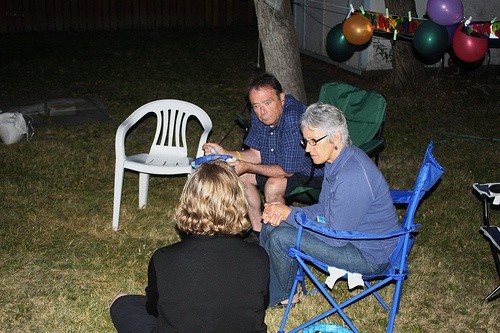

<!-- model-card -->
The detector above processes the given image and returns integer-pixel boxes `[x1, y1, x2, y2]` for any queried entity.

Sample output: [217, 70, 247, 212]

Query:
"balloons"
[452, 24, 488, 62]
[414, 20, 448, 57]
[326, 23, 356, 62]
[427, 0, 463, 26]
[343, 15, 373, 45]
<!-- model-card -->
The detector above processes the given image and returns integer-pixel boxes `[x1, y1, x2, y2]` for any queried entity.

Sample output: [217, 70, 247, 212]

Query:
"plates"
[191, 155, 240, 170]
[302, 323, 355, 333]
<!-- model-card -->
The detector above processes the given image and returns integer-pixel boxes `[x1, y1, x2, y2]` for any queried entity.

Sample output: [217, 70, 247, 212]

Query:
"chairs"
[111, 98, 213, 233]
[220, 80, 389, 240]
[472, 182, 500, 301]
[278, 140, 445, 333]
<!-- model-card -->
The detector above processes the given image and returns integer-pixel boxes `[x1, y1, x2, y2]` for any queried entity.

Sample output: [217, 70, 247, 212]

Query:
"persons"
[109, 159, 270, 333]
[259, 102, 403, 308]
[202, 74, 324, 233]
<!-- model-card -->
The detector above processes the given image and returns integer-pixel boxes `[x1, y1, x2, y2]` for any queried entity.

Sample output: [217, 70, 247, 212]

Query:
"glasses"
[300, 134, 327, 146]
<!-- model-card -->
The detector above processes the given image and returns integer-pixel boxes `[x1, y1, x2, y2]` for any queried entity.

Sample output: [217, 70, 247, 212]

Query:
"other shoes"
[273, 299, 300, 309]
